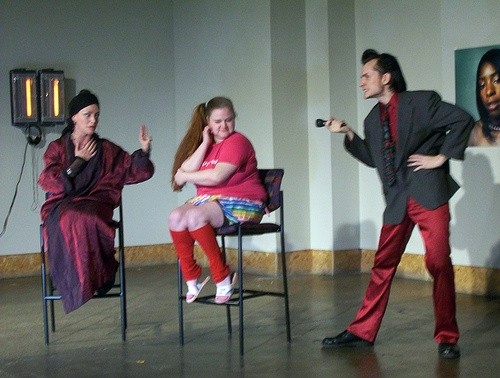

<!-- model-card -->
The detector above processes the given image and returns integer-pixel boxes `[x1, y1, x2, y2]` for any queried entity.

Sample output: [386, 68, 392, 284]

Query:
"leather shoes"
[438, 343, 459, 360]
[320, 329, 374, 348]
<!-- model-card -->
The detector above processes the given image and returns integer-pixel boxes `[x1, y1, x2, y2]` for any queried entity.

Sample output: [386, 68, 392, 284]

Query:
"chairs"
[38, 188, 128, 345]
[177, 167, 293, 359]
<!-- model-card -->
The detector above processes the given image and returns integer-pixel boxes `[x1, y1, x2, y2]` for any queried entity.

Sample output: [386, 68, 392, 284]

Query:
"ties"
[383, 103, 396, 188]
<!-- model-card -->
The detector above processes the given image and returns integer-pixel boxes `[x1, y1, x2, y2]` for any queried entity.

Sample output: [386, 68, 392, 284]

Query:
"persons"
[320, 48, 479, 359]
[167, 95, 267, 306]
[35, 90, 155, 316]
[464, 47, 500, 148]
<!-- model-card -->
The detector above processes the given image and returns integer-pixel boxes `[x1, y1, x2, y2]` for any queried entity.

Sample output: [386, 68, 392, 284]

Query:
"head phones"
[24, 125, 42, 146]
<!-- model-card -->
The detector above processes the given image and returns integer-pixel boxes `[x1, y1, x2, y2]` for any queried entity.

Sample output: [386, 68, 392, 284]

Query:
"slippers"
[214, 271, 238, 303]
[185, 275, 210, 304]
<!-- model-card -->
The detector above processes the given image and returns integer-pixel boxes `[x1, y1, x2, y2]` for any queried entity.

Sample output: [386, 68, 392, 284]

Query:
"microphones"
[316, 118, 347, 127]
